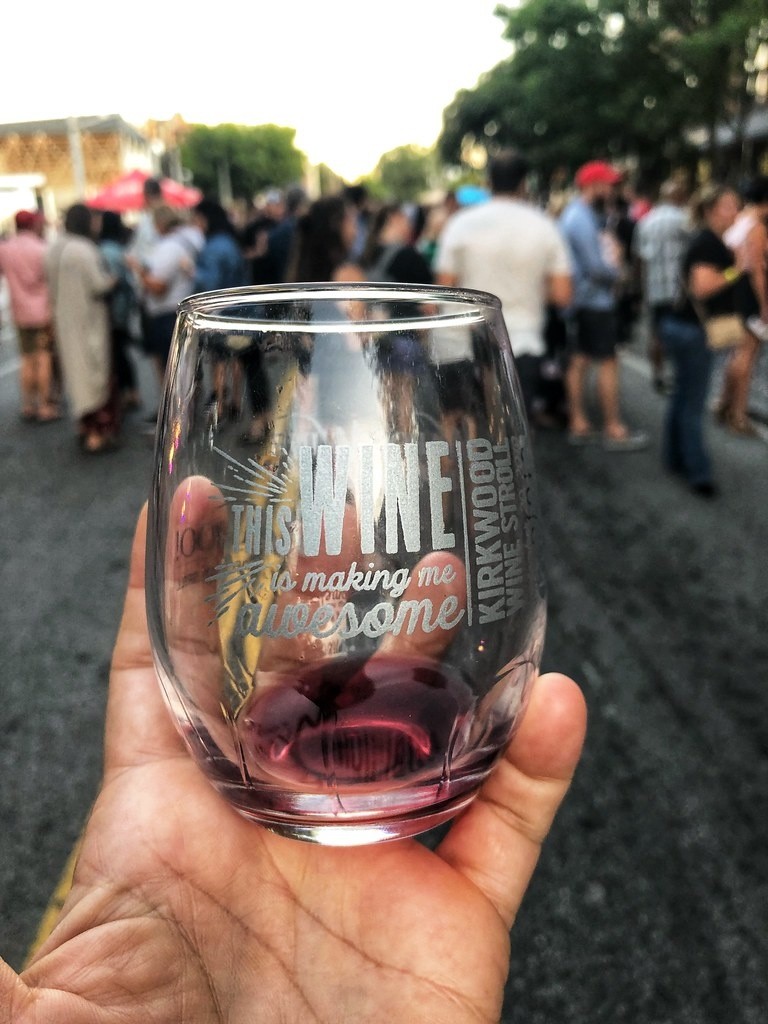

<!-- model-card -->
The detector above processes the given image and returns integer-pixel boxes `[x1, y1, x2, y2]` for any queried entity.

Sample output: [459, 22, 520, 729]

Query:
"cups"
[144, 280, 546, 848]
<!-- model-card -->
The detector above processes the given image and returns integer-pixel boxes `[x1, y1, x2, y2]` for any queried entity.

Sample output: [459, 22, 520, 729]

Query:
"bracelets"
[723, 266, 738, 282]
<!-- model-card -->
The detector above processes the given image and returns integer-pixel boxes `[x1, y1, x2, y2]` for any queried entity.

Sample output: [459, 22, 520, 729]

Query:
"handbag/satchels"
[702, 316, 747, 347]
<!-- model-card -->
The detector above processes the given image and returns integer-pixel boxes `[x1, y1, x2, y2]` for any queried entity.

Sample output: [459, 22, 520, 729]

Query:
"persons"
[1, 473, 590, 1024]
[0, 146, 768, 500]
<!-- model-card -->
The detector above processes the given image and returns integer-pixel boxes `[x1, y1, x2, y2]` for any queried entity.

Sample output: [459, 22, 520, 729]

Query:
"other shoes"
[608, 432, 647, 451]
[568, 431, 583, 445]
[20, 380, 270, 453]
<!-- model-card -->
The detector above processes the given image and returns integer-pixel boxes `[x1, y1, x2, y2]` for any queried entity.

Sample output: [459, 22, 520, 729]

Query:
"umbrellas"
[86, 170, 204, 212]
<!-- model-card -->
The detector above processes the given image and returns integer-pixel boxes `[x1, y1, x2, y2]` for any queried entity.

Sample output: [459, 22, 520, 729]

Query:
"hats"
[16, 213, 36, 225]
[576, 163, 620, 185]
